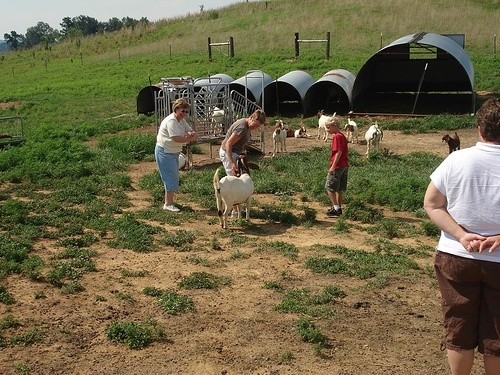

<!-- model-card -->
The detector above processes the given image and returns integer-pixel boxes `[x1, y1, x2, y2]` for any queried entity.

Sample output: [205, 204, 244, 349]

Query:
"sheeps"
[317, 110, 336, 142]
[364, 125, 384, 153]
[212, 149, 255, 230]
[208, 105, 237, 138]
[271, 119, 315, 158]
[344, 120, 360, 144]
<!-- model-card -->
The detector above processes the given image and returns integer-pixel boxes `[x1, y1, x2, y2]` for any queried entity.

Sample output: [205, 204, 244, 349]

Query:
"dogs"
[442, 132, 460, 153]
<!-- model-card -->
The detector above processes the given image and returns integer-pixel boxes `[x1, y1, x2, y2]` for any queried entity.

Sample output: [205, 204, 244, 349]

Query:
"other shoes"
[326, 208, 342, 215]
[163, 203, 181, 212]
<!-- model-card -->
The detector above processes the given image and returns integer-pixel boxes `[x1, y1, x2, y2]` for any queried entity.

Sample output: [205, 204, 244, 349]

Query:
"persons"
[155, 98, 195, 212]
[325, 117, 348, 217]
[219, 109, 266, 219]
[424, 98, 500, 375]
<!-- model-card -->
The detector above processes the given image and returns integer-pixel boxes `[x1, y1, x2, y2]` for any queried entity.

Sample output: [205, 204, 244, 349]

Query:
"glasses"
[179, 109, 188, 114]
